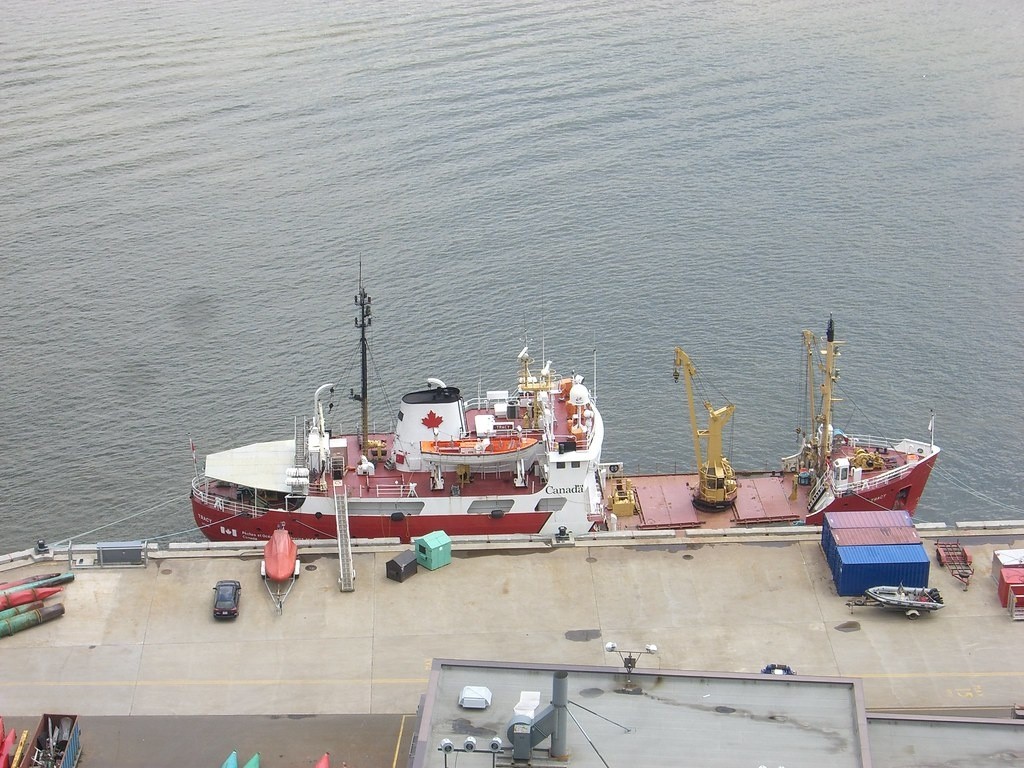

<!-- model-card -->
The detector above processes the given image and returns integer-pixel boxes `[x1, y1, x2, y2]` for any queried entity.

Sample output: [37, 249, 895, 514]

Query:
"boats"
[419, 438, 540, 467]
[191, 254, 943, 540]
[264, 529, 298, 581]
[865, 578, 946, 612]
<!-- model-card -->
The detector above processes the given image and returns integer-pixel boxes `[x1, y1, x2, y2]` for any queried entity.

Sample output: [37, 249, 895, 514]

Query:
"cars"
[213, 579, 242, 620]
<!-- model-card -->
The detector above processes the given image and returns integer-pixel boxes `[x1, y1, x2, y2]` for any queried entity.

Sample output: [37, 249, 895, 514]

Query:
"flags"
[190, 440, 197, 463]
[928, 420, 932, 434]
